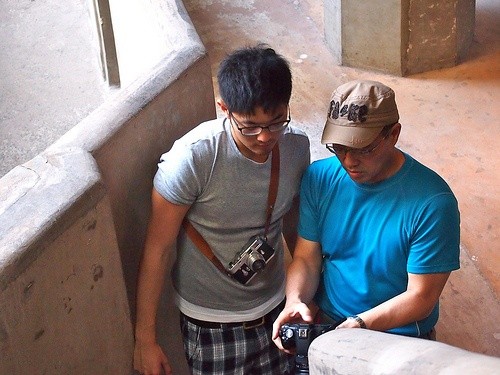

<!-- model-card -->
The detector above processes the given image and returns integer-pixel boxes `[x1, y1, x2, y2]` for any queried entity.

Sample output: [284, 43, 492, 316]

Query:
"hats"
[321, 80, 399, 148]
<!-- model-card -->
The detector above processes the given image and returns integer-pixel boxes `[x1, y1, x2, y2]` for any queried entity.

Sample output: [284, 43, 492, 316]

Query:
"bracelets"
[352, 315, 367, 329]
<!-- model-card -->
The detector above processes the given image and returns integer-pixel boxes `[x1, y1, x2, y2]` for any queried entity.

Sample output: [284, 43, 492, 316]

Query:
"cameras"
[279, 323, 336, 375]
[226, 235, 276, 286]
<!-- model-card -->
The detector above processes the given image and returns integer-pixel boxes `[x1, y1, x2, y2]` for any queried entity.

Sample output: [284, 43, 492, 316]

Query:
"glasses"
[325, 134, 385, 156]
[227, 104, 291, 135]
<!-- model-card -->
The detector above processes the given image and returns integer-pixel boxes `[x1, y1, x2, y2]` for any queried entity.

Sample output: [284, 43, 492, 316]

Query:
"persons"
[134, 41, 319, 375]
[272, 79, 460, 355]
